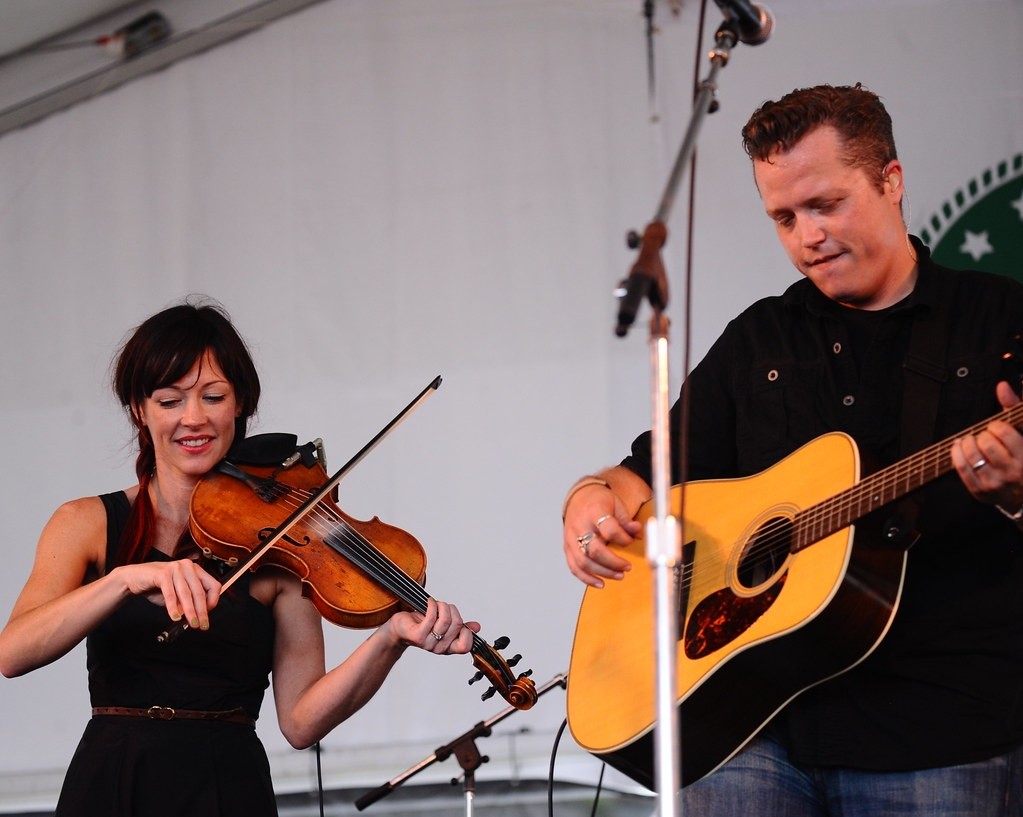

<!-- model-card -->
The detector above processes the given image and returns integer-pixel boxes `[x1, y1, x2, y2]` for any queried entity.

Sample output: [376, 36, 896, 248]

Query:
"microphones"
[715, 0, 773, 47]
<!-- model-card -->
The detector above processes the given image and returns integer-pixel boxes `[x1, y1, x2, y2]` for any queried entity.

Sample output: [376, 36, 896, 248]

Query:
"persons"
[0, 304, 480, 817]
[563, 84, 1023, 817]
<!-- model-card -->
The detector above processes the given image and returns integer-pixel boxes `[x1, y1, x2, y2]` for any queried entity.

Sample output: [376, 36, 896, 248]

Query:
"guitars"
[566, 401, 1022, 795]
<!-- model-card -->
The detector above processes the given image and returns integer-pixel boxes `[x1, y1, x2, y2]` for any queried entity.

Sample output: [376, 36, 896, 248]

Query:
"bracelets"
[562, 479, 612, 524]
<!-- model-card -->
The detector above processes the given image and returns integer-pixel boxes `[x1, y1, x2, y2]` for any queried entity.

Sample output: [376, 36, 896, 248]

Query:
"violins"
[186, 432, 539, 712]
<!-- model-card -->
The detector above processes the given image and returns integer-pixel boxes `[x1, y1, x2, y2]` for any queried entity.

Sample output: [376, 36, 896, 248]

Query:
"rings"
[577, 533, 597, 554]
[430, 630, 444, 640]
[972, 460, 986, 470]
[596, 515, 611, 527]
